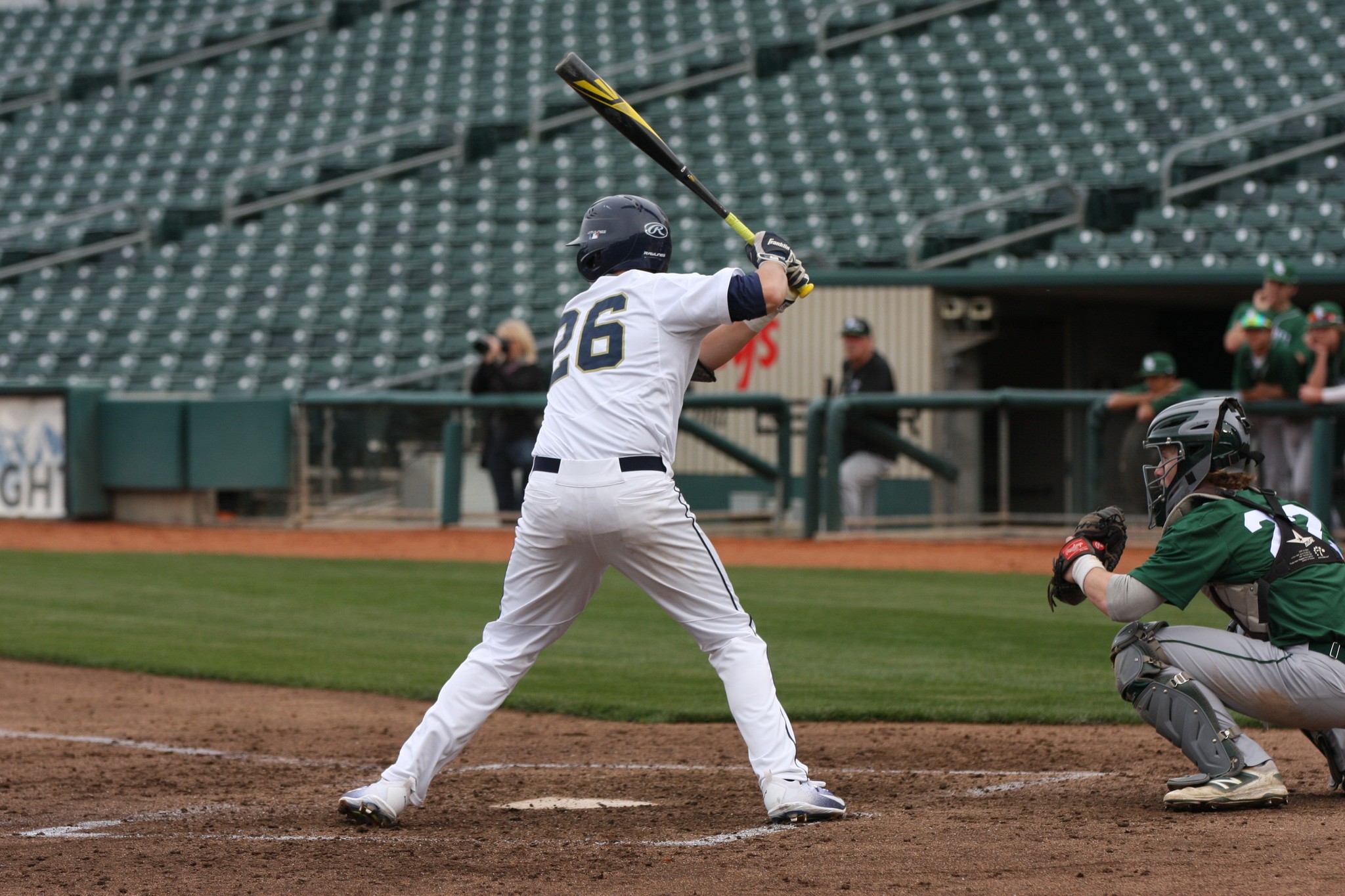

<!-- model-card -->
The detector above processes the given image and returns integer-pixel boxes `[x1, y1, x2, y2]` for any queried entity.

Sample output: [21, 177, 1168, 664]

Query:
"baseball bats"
[553, 50, 816, 300]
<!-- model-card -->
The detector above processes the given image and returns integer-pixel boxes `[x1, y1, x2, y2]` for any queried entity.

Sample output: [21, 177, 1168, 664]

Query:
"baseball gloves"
[1052, 505, 1129, 606]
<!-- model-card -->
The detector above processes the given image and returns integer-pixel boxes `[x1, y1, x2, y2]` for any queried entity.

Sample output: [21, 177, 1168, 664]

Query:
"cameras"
[474, 338, 511, 356]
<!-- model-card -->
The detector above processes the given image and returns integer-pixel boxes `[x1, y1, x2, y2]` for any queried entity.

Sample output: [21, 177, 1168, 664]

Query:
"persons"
[1063, 396, 1345, 809]
[469, 318, 542, 526]
[819, 315, 901, 533]
[1087, 258, 1345, 511]
[336, 194, 847, 828]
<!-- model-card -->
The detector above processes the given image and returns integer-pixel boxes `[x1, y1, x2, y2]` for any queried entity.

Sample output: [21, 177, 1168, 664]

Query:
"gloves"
[745, 232, 790, 271]
[742, 257, 808, 334]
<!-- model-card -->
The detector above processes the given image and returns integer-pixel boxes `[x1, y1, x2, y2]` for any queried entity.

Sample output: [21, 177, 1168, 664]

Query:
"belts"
[532, 456, 668, 474]
[1309, 640, 1345, 663]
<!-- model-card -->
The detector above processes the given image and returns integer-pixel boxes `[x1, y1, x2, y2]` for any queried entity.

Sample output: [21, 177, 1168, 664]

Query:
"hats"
[1242, 312, 1270, 330]
[842, 319, 872, 336]
[1264, 259, 1300, 286]
[1304, 301, 1344, 329]
[1140, 352, 1173, 377]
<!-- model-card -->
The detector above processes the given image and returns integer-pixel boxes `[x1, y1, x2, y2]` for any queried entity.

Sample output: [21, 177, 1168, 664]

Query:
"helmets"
[564, 194, 671, 281]
[1140, 397, 1251, 530]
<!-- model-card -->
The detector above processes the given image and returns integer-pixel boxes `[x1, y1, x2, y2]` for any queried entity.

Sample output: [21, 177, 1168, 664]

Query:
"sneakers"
[761, 769, 847, 822]
[1163, 759, 1289, 811]
[337, 778, 418, 828]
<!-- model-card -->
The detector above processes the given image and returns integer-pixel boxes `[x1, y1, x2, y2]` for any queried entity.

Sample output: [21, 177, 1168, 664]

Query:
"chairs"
[0, 0, 1345, 397]
